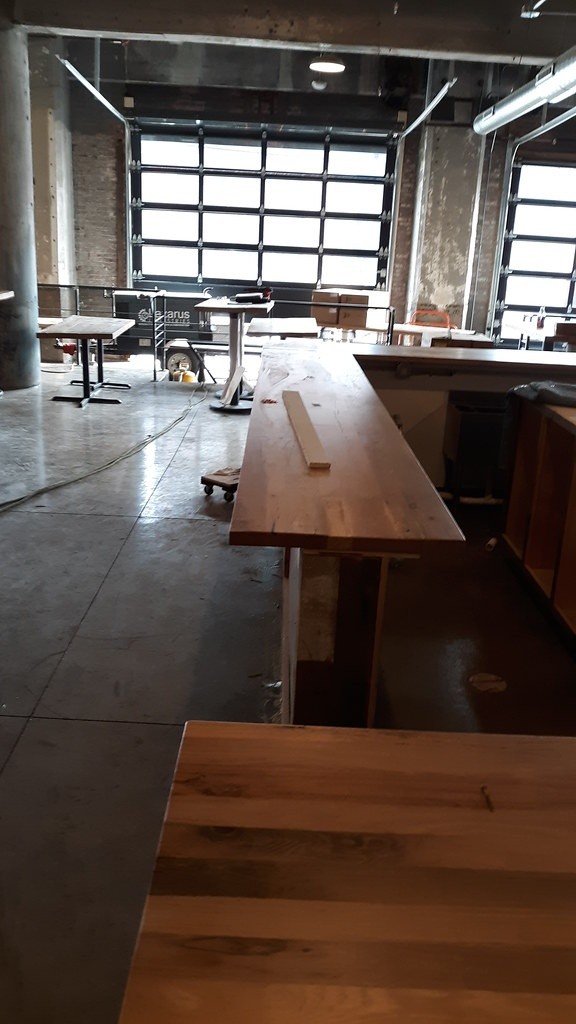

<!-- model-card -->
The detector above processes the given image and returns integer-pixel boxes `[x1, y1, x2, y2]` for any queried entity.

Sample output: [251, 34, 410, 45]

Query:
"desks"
[121, 722, 576, 1024]
[36, 314, 136, 408]
[246, 318, 318, 340]
[195, 296, 276, 415]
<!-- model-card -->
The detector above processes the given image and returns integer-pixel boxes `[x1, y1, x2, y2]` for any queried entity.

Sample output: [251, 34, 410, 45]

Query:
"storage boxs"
[311, 288, 390, 328]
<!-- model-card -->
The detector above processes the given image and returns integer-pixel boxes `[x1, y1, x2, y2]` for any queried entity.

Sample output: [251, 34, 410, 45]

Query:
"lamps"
[310, 52, 346, 73]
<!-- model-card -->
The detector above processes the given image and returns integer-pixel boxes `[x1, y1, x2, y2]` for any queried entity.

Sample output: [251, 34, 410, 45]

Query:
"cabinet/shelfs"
[500, 397, 576, 643]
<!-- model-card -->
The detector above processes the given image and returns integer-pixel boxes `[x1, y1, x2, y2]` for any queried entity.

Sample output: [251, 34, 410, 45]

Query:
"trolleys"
[398, 310, 458, 347]
[200, 467, 240, 502]
